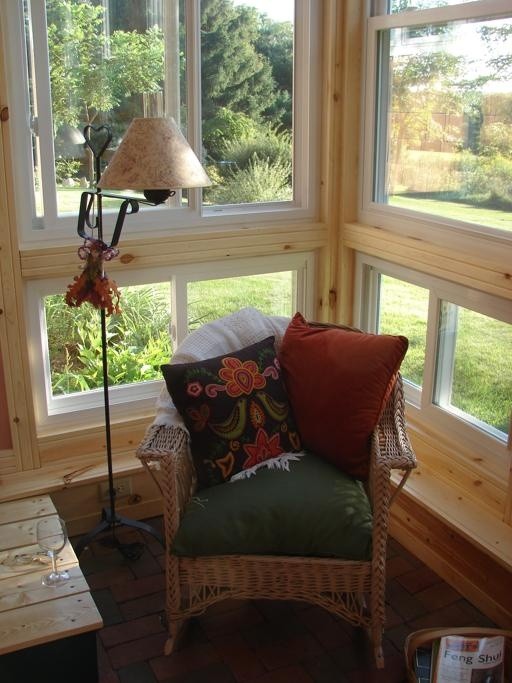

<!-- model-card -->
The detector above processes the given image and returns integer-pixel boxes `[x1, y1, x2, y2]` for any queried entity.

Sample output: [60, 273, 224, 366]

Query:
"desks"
[2, 494, 105, 683]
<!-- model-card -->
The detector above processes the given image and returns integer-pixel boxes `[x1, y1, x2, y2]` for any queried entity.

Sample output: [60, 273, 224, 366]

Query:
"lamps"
[72, 114, 165, 565]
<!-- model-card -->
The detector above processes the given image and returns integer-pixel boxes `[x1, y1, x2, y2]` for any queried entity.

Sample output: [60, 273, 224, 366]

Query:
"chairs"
[133, 318, 416, 671]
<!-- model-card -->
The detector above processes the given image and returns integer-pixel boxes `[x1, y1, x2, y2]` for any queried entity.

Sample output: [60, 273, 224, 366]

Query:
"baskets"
[405, 625, 512, 683]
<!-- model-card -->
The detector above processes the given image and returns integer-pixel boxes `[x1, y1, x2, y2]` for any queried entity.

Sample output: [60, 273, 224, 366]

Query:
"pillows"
[160, 336, 304, 491]
[169, 448, 374, 560]
[277, 313, 409, 482]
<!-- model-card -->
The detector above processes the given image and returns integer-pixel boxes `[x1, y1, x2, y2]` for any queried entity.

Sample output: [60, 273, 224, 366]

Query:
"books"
[429, 635, 505, 683]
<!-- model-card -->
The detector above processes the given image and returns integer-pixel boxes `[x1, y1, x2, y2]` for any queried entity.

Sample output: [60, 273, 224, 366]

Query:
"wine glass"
[37, 517, 70, 587]
